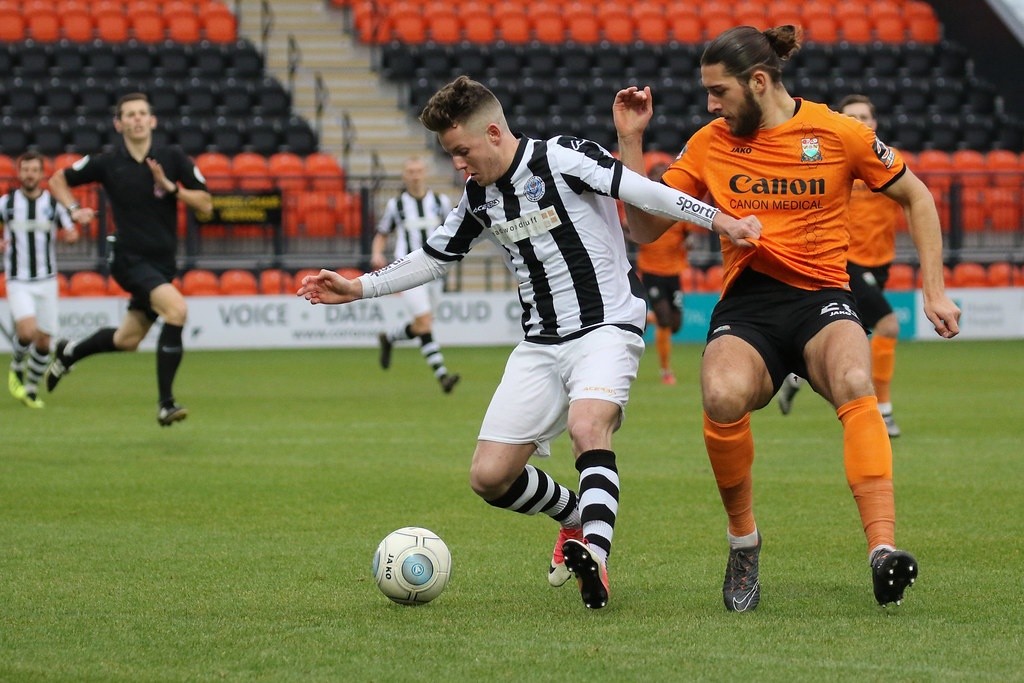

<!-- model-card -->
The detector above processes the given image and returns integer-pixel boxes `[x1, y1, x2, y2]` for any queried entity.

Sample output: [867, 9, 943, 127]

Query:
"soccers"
[372, 527, 453, 607]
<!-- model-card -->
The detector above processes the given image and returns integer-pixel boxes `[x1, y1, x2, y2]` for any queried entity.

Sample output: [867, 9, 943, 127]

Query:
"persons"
[638, 161, 691, 386]
[0, 152, 79, 409]
[47, 91, 211, 427]
[297, 76, 764, 618]
[614, 24, 961, 610]
[370, 157, 461, 392]
[778, 94, 901, 437]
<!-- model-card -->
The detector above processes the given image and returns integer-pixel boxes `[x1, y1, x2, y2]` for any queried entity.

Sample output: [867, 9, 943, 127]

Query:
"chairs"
[1, 0, 1024, 297]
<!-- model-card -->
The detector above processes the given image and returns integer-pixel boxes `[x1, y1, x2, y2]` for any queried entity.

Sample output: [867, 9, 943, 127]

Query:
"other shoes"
[443, 374, 461, 392]
[884, 418, 899, 436]
[378, 332, 391, 369]
[777, 375, 800, 414]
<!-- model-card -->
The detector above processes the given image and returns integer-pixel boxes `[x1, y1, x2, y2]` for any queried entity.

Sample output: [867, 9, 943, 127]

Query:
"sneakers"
[722, 531, 761, 613]
[157, 405, 188, 426]
[7, 369, 26, 400]
[562, 539, 609, 609]
[22, 394, 43, 409]
[869, 549, 918, 608]
[548, 525, 584, 587]
[45, 338, 70, 392]
[662, 372, 676, 385]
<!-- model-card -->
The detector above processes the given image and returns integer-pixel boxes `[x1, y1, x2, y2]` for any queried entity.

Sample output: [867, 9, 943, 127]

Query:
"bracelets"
[167, 185, 178, 196]
[66, 203, 80, 214]
